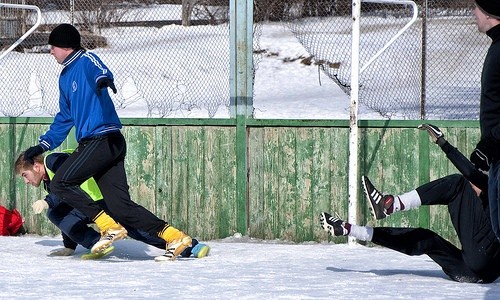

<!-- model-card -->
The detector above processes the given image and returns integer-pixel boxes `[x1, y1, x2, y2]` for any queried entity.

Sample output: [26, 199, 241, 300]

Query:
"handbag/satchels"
[0, 206, 22, 237]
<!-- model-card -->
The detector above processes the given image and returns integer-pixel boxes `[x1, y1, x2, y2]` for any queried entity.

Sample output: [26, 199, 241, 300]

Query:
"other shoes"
[191, 243, 210, 258]
[81, 245, 114, 259]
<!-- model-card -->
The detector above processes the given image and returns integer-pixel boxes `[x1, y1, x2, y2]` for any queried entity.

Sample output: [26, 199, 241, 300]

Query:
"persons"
[23, 24, 192, 261]
[470, 0, 500, 242]
[15, 149, 210, 259]
[320, 123, 500, 284]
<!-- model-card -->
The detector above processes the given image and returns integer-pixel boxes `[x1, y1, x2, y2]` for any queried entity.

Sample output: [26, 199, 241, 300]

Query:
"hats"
[474, 0, 500, 20]
[48, 23, 80, 48]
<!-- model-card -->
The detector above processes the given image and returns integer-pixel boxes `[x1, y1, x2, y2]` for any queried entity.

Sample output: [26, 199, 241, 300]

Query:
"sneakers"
[90, 222, 128, 254]
[362, 175, 394, 219]
[154, 231, 192, 261]
[320, 212, 350, 236]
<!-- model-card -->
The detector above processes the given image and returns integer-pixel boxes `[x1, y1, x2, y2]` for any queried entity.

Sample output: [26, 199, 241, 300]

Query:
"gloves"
[51, 247, 74, 256]
[470, 140, 496, 173]
[32, 199, 49, 214]
[418, 124, 445, 144]
[23, 145, 45, 165]
[96, 77, 118, 96]
